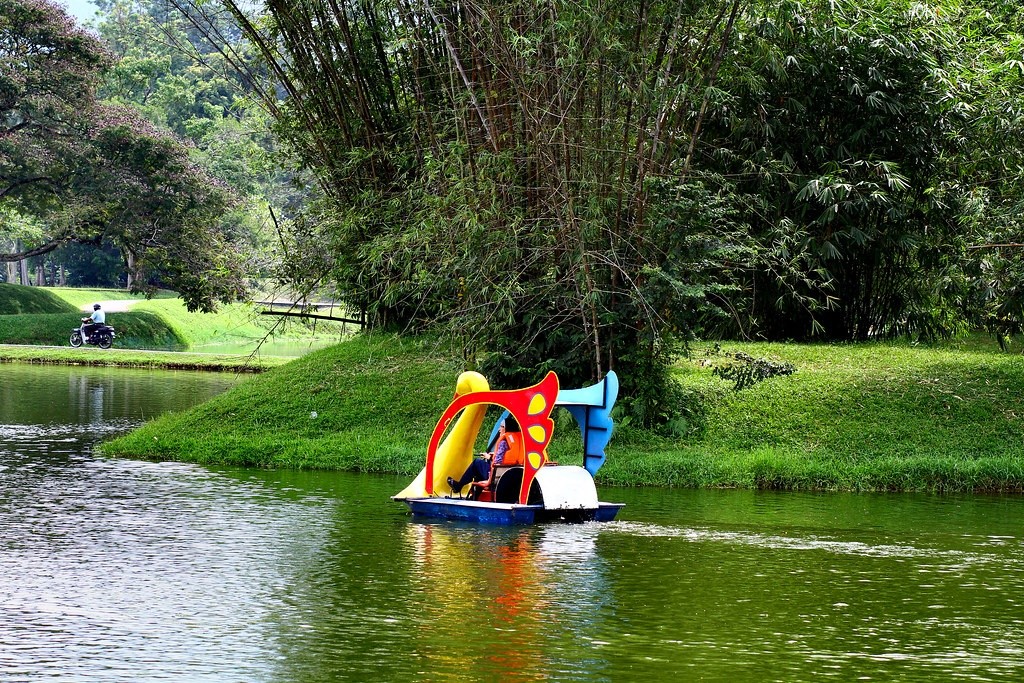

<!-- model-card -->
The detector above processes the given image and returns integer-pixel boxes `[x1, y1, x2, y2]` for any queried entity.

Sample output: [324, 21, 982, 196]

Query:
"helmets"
[93, 304, 101, 309]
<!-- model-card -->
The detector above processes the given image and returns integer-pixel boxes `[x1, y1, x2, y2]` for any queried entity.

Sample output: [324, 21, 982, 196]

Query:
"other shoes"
[447, 476, 460, 493]
[470, 495, 478, 501]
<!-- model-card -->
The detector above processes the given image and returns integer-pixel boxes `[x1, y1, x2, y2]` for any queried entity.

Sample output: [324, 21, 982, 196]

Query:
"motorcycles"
[69, 317, 116, 349]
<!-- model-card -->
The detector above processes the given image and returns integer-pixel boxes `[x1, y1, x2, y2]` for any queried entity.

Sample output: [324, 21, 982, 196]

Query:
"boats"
[390, 370, 626, 527]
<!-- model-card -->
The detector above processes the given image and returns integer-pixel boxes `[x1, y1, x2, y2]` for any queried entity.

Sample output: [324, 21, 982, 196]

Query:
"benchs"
[473, 461, 559, 502]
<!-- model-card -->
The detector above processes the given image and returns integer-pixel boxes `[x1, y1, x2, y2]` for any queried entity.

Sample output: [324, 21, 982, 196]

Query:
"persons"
[446, 417, 524, 494]
[81, 303, 106, 344]
